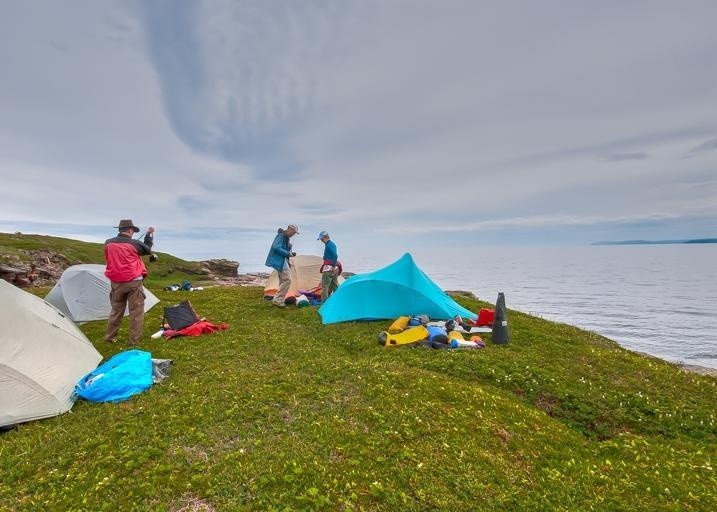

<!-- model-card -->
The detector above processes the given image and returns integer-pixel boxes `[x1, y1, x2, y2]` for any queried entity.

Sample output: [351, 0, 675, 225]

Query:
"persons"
[101, 217, 155, 344]
[264, 222, 301, 309]
[315, 230, 342, 306]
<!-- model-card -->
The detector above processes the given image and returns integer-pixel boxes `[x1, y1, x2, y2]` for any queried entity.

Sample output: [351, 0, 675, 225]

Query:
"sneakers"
[272, 300, 285, 307]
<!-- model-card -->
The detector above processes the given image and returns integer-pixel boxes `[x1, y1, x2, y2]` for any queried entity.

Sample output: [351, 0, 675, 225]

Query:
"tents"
[262, 253, 345, 297]
[314, 251, 480, 327]
[0, 275, 105, 431]
[40, 263, 161, 323]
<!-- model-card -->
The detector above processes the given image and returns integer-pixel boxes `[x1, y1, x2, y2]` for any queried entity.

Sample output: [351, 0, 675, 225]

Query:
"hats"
[316, 231, 328, 241]
[288, 224, 300, 234]
[113, 220, 139, 232]
